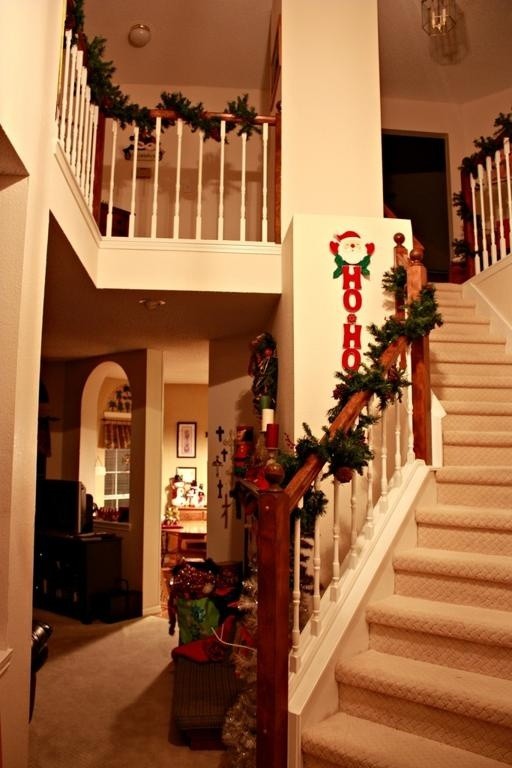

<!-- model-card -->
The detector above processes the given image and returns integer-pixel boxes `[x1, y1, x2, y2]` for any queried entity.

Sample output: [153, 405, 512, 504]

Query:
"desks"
[162, 526, 208, 566]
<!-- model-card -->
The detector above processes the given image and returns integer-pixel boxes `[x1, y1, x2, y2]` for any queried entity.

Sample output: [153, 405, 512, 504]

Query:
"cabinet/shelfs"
[34, 536, 122, 625]
[100, 202, 131, 236]
[171, 505, 209, 520]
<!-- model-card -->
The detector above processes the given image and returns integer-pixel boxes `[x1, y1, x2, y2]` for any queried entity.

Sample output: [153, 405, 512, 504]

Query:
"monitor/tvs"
[34, 480, 87, 535]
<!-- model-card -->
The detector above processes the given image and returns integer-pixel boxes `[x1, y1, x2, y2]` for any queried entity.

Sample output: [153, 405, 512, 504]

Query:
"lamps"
[128, 24, 152, 49]
[421, 0, 458, 36]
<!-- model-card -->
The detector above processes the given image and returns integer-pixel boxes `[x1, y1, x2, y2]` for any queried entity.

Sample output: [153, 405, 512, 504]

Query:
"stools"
[30, 615, 53, 725]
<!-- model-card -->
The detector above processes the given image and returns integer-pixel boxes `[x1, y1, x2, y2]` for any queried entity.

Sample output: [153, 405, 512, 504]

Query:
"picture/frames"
[176, 421, 197, 459]
[176, 466, 198, 485]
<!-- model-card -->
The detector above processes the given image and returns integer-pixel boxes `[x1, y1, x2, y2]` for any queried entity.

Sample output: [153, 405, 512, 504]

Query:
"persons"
[252, 340, 278, 407]
[231, 429, 253, 478]
